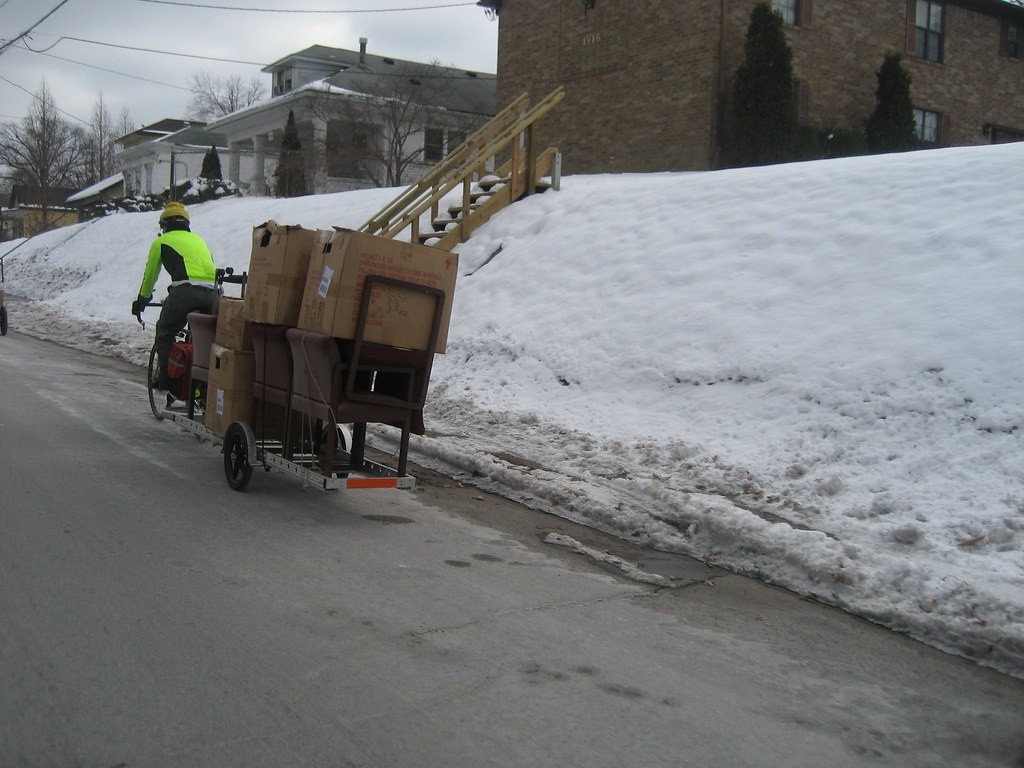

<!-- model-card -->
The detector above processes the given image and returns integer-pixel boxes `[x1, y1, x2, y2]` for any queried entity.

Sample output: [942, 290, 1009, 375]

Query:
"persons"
[133, 203, 221, 391]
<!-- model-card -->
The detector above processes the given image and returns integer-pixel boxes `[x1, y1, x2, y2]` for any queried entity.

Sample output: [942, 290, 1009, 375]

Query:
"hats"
[160, 202, 191, 226]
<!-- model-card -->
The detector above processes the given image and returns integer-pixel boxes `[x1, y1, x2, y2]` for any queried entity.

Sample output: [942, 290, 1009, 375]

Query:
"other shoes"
[149, 380, 171, 391]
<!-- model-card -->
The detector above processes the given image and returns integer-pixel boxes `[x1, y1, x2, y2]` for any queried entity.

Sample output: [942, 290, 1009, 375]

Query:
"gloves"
[132, 294, 153, 315]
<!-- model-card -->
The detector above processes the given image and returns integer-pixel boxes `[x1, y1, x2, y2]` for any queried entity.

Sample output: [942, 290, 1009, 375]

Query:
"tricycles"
[134, 266, 439, 493]
